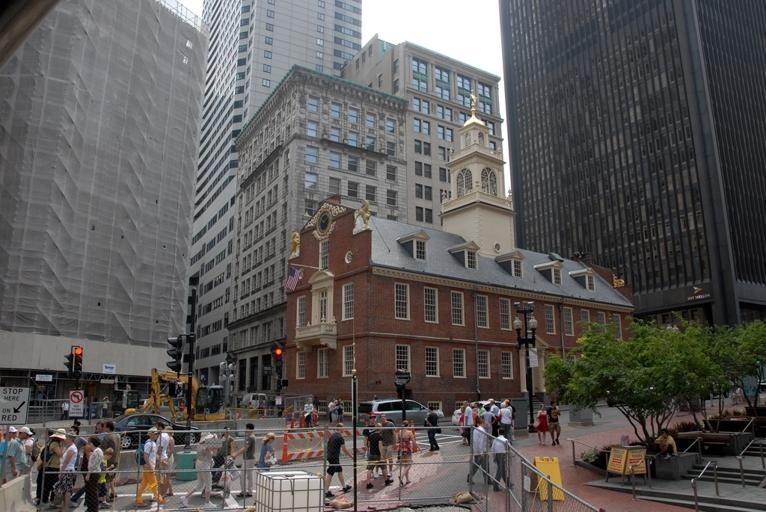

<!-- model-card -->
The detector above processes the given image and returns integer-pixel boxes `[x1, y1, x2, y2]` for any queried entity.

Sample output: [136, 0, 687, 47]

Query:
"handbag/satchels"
[155, 445, 162, 469]
[435, 425, 442, 434]
[265, 448, 279, 466]
[217, 459, 240, 487]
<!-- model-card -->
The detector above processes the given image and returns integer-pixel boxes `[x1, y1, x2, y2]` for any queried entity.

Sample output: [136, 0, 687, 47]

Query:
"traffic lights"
[166, 337, 183, 374]
[274, 348, 282, 376]
[64, 353, 74, 370]
[72, 347, 82, 378]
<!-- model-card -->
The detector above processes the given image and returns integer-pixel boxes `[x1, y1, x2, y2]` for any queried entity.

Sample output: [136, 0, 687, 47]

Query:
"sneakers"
[236, 491, 252, 497]
[324, 446, 439, 499]
[134, 502, 149, 507]
[97, 493, 118, 509]
[179, 496, 190, 508]
[202, 502, 217, 508]
[150, 492, 174, 504]
[47, 502, 56, 509]
[69, 499, 79, 507]
[462, 438, 561, 493]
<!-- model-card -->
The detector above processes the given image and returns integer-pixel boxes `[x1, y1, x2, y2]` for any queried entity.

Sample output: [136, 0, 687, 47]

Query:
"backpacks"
[39, 442, 56, 463]
[29, 438, 39, 463]
[134, 441, 153, 465]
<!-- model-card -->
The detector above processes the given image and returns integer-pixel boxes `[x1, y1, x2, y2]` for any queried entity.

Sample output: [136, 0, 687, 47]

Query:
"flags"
[285, 267, 301, 291]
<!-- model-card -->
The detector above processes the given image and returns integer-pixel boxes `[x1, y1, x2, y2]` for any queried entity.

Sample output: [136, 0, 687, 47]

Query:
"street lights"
[391, 371, 410, 423]
[512, 298, 541, 430]
[218, 361, 237, 422]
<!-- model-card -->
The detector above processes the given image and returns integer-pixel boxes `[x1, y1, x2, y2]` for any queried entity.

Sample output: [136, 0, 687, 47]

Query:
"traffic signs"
[0, 386, 30, 425]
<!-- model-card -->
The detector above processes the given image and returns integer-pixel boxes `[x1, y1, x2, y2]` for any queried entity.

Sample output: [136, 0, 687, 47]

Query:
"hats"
[8, 425, 18, 434]
[198, 430, 214, 444]
[147, 426, 160, 435]
[163, 425, 175, 436]
[48, 428, 67, 440]
[19, 426, 33, 435]
[262, 432, 275, 441]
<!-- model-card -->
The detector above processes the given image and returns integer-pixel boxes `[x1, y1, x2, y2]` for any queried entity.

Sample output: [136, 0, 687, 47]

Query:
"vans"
[136, 398, 149, 409]
[239, 392, 268, 408]
[358, 398, 434, 426]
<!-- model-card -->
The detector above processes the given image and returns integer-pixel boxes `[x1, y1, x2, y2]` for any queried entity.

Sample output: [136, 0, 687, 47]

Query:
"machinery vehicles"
[121, 367, 229, 421]
[109, 389, 177, 421]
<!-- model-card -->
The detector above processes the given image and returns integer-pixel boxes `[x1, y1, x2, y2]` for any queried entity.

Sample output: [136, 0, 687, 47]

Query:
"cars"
[604, 381, 729, 406]
[110, 412, 201, 448]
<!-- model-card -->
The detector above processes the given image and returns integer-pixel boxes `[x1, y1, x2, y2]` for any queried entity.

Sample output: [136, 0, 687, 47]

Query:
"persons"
[363, 412, 423, 489]
[547, 399, 561, 445]
[303, 397, 314, 428]
[451, 396, 516, 491]
[59, 400, 69, 421]
[324, 422, 354, 497]
[328, 397, 344, 423]
[470, 89, 478, 108]
[423, 406, 440, 451]
[0, 419, 277, 511]
[102, 395, 110, 419]
[313, 396, 320, 411]
[247, 392, 278, 417]
[533, 403, 549, 445]
[654, 427, 679, 458]
